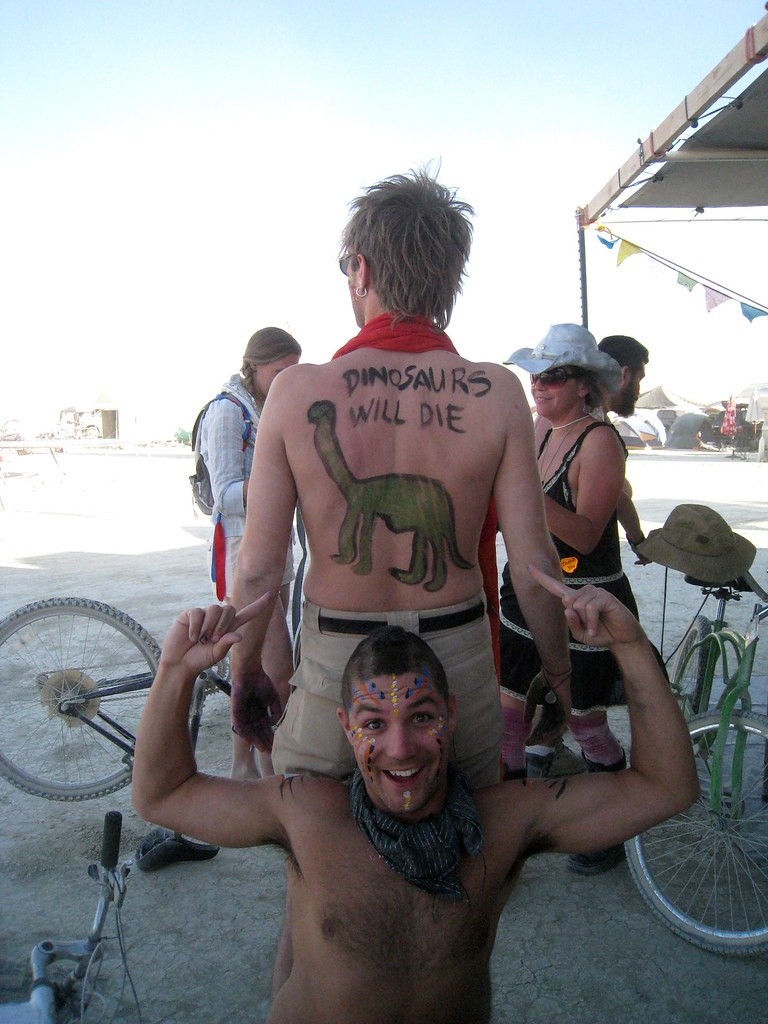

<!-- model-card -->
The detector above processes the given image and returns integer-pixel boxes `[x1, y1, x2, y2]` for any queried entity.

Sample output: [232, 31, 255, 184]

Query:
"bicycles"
[0, 811, 144, 1024]
[626, 575, 768, 957]
[0, 598, 231, 872]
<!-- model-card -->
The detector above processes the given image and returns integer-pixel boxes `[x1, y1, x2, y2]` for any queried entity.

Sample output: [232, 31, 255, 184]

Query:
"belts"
[319, 598, 485, 635]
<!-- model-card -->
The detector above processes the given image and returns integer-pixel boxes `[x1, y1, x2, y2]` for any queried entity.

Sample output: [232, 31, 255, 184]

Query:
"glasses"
[530, 368, 583, 389]
[339, 253, 357, 277]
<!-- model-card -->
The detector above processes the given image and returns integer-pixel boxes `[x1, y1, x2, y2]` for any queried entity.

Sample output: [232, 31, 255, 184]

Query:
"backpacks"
[186, 391, 253, 519]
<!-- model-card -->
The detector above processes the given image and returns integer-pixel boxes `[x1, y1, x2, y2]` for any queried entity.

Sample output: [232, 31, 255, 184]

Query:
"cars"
[712, 409, 764, 449]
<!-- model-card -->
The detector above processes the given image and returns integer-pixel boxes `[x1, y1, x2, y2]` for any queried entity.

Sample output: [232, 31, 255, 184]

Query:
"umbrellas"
[745, 390, 765, 434]
[720, 395, 738, 443]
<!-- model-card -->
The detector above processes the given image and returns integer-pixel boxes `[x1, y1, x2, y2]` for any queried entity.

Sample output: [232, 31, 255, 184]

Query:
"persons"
[498, 324, 627, 780]
[695, 427, 720, 452]
[230, 157, 572, 1024]
[198, 326, 302, 781]
[523, 335, 668, 739]
[130, 564, 698, 1024]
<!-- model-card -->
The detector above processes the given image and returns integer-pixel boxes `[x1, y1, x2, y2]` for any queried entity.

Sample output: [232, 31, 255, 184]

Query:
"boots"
[565, 746, 627, 875]
[503, 755, 528, 782]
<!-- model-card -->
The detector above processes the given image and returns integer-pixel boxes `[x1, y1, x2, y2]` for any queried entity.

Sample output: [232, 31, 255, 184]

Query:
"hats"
[635, 504, 756, 584]
[502, 323, 622, 396]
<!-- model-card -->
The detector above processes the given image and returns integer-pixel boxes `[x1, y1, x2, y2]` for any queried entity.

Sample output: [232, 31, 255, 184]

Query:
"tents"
[610, 383, 768, 462]
